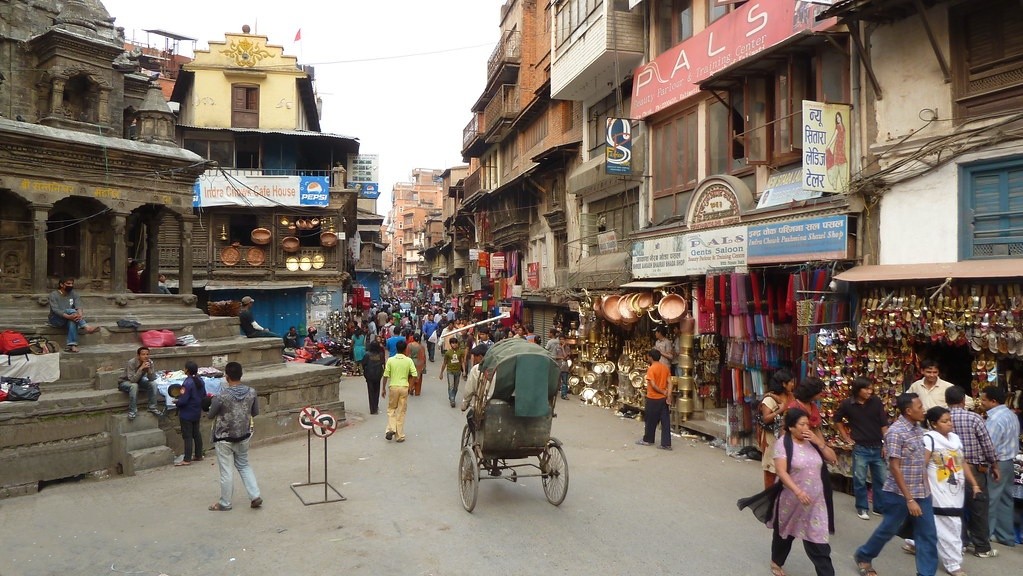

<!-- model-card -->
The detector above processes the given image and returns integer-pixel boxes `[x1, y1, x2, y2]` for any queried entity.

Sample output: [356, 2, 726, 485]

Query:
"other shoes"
[962, 546, 968, 555]
[410, 389, 414, 395]
[251, 499, 263, 508]
[396, 438, 405, 442]
[872, 511, 883, 517]
[451, 402, 455, 408]
[657, 445, 672, 450]
[430, 359, 434, 362]
[946, 569, 968, 576]
[386, 431, 395, 440]
[901, 544, 916, 555]
[449, 397, 451, 401]
[208, 503, 232, 511]
[974, 549, 999, 558]
[857, 510, 870, 520]
[561, 395, 569, 400]
[635, 439, 650, 446]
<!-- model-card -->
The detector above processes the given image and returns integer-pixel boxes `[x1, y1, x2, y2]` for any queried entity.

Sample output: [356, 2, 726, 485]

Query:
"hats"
[242, 296, 254, 304]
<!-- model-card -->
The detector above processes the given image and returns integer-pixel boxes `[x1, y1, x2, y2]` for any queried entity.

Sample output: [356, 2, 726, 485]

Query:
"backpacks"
[0, 329, 28, 354]
[366, 356, 384, 382]
[382, 324, 392, 339]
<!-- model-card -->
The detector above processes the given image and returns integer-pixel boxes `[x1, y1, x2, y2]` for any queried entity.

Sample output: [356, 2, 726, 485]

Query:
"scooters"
[281, 342, 342, 366]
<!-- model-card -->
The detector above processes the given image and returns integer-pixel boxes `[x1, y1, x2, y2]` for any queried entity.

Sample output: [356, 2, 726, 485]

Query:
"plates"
[221, 245, 241, 265]
[286, 253, 324, 272]
[246, 246, 266, 266]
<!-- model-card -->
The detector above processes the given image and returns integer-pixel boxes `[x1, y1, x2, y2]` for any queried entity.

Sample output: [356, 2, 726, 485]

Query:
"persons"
[158, 275, 171, 295]
[736, 356, 1020, 576]
[652, 326, 673, 369]
[283, 326, 301, 349]
[361, 341, 384, 415]
[439, 338, 466, 409]
[635, 349, 674, 451]
[207, 363, 263, 511]
[673, 326, 681, 376]
[382, 340, 418, 442]
[48, 276, 101, 352]
[239, 296, 280, 338]
[171, 361, 206, 466]
[303, 327, 318, 363]
[127, 257, 147, 294]
[461, 344, 497, 432]
[348, 283, 572, 417]
[117, 346, 163, 420]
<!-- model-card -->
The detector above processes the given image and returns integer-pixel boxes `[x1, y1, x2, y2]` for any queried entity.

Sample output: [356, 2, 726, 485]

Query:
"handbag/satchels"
[7, 381, 41, 401]
[29, 340, 60, 355]
[428, 330, 437, 344]
[754, 394, 783, 440]
[140, 329, 176, 348]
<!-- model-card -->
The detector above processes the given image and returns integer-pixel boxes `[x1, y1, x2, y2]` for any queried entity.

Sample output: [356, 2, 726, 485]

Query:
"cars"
[399, 303, 412, 314]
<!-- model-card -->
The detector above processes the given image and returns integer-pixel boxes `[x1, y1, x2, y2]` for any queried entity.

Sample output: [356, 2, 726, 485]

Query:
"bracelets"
[819, 443, 826, 452]
[796, 490, 803, 496]
[773, 411, 776, 416]
[907, 499, 914, 502]
[971, 484, 980, 487]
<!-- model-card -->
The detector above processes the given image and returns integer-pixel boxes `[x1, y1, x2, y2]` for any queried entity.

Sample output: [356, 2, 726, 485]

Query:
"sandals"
[854, 556, 878, 576]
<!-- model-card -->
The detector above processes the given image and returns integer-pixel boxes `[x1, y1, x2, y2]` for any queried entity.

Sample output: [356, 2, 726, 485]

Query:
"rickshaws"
[458, 339, 569, 513]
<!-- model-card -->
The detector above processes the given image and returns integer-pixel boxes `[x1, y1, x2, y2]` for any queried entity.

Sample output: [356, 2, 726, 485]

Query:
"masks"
[62, 283, 74, 292]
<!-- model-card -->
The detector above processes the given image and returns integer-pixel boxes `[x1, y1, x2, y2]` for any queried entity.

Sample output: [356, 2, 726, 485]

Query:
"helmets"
[167, 384, 182, 398]
[308, 327, 317, 333]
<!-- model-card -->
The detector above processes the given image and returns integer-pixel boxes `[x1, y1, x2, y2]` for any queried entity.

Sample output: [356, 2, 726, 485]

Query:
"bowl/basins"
[251, 228, 272, 244]
[282, 237, 299, 252]
[601, 292, 689, 324]
[320, 232, 338, 246]
[569, 360, 648, 407]
[296, 217, 319, 229]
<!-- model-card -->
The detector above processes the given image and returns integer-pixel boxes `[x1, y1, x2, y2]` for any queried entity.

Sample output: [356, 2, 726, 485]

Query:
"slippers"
[128, 413, 135, 419]
[175, 457, 203, 466]
[147, 408, 163, 417]
[78, 327, 100, 335]
[769, 563, 786, 576]
[64, 346, 80, 352]
